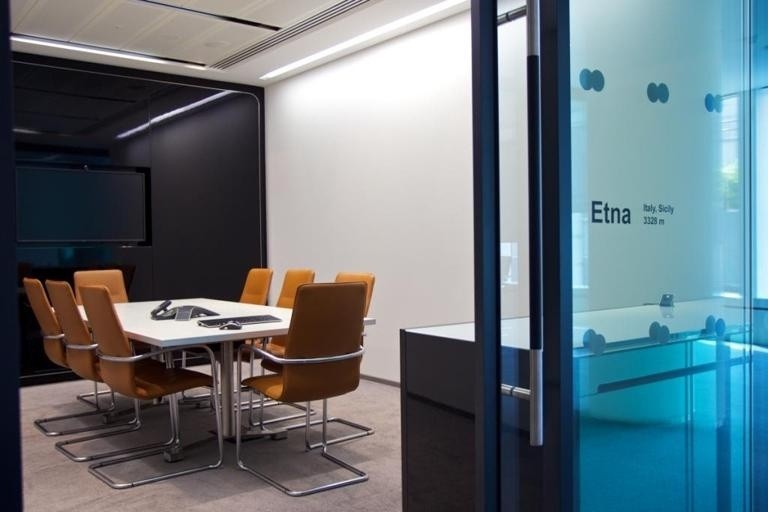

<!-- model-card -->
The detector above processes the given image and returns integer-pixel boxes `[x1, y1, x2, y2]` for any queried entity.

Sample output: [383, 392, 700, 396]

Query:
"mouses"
[220, 320, 241, 329]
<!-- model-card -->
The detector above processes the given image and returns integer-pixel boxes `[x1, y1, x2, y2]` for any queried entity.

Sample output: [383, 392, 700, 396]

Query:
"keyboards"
[198, 314, 282, 328]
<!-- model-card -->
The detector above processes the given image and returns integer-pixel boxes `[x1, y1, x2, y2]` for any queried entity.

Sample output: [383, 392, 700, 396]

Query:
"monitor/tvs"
[15, 158, 152, 250]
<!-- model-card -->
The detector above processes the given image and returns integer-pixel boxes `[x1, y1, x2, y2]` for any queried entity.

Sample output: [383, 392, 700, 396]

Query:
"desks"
[46, 297, 375, 445]
[400, 297, 729, 509]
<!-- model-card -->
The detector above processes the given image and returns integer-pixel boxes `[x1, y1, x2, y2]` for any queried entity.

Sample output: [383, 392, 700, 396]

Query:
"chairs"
[70, 270, 163, 412]
[46, 280, 179, 462]
[235, 283, 371, 496]
[236, 269, 318, 428]
[23, 276, 139, 439]
[79, 285, 224, 489]
[259, 272, 374, 449]
[178, 268, 274, 408]
